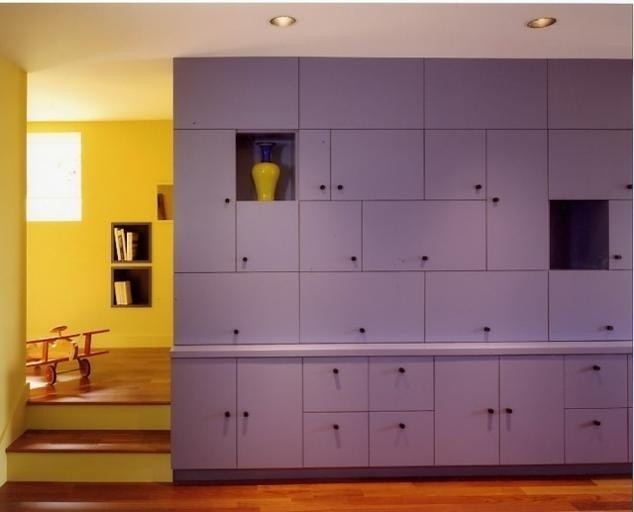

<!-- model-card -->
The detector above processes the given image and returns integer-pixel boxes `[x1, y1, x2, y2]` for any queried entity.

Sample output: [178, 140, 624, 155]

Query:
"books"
[113, 227, 139, 306]
[158, 193, 166, 220]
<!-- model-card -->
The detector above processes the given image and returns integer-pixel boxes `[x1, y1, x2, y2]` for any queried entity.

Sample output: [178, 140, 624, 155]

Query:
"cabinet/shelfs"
[435, 354, 565, 480]
[304, 357, 435, 482]
[168, 357, 304, 485]
[172, 56, 300, 346]
[109, 218, 154, 310]
[298, 56, 631, 344]
[567, 354, 634, 476]
